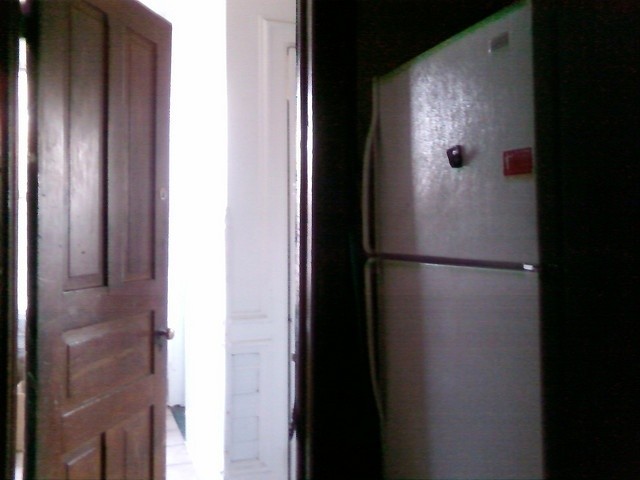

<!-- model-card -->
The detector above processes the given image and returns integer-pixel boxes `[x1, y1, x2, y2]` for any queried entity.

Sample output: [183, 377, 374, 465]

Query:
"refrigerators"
[361, 0, 640, 479]
